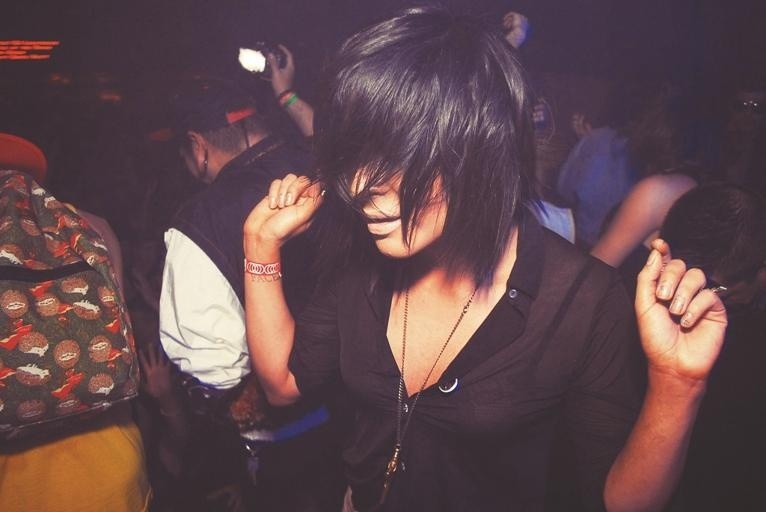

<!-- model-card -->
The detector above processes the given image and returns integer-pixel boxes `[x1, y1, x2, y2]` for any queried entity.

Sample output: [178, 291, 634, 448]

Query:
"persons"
[5, 1, 764, 512]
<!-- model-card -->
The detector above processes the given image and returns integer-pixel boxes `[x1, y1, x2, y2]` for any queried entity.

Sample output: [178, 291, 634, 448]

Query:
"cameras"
[237, 39, 285, 77]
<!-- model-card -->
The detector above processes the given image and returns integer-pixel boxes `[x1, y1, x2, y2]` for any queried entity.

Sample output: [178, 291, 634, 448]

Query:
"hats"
[150, 73, 258, 143]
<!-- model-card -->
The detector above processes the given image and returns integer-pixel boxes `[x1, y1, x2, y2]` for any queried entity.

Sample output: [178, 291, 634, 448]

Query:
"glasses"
[730, 99, 766, 116]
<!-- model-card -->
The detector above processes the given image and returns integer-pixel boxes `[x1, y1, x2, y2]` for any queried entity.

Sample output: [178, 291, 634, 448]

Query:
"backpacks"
[1, 170, 143, 443]
